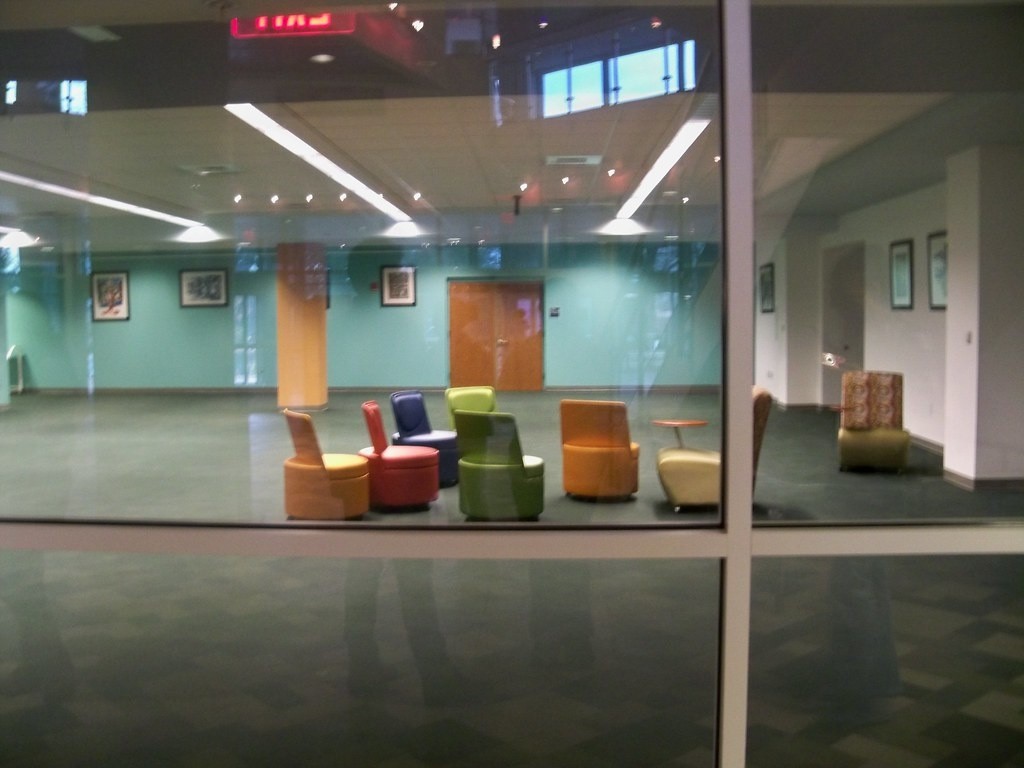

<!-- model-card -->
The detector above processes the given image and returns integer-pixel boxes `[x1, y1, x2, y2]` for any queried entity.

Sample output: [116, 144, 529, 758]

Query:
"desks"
[653, 419, 709, 447]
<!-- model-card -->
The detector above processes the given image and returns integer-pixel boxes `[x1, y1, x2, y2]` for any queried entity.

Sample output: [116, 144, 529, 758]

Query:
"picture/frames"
[889, 238, 914, 310]
[927, 230, 947, 311]
[758, 264, 776, 313]
[179, 268, 229, 308]
[90, 270, 130, 322]
[380, 264, 416, 307]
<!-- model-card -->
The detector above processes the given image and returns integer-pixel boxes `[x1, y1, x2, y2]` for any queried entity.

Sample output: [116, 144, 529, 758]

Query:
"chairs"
[444, 385, 499, 431]
[453, 408, 544, 522]
[358, 399, 441, 512]
[559, 398, 641, 503]
[283, 408, 370, 521]
[390, 388, 460, 487]
[836, 371, 911, 475]
[657, 385, 772, 513]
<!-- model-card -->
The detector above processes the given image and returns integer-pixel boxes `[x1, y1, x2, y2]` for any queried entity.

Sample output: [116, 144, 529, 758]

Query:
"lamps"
[0, 150, 91, 200]
[223, 101, 417, 221]
[85, 180, 206, 228]
[615, 93, 720, 218]
